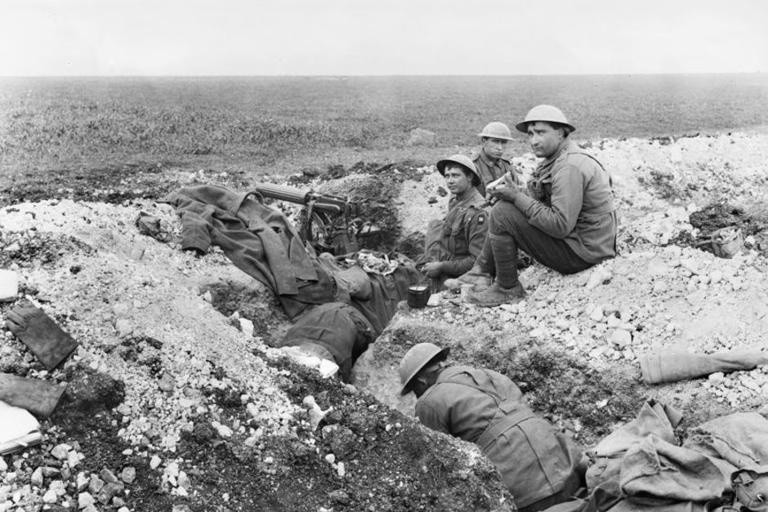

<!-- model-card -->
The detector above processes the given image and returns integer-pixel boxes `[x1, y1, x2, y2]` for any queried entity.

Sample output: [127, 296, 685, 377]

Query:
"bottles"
[408, 285, 429, 308]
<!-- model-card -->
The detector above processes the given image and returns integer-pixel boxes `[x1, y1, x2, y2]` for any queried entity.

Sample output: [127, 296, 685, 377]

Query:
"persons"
[400, 341, 589, 512]
[474, 120, 514, 184]
[426, 153, 491, 292]
[445, 104, 619, 305]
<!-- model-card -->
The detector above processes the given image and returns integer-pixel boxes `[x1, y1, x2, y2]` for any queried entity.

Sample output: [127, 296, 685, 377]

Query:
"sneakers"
[444, 270, 493, 291]
[469, 283, 526, 306]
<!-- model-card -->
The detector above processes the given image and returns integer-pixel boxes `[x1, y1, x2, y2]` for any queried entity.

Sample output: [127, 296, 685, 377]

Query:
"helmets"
[478, 122, 513, 141]
[516, 105, 575, 136]
[437, 154, 481, 185]
[399, 343, 450, 396]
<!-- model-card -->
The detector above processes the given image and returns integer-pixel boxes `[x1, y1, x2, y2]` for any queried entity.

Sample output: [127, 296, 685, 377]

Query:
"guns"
[485, 171, 513, 191]
[255, 183, 360, 253]
